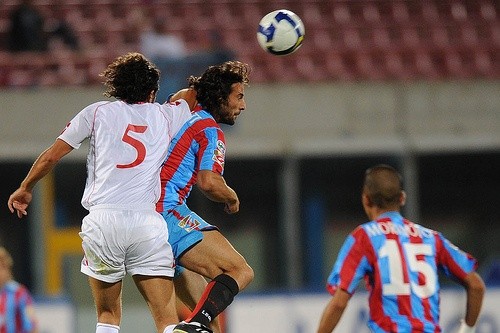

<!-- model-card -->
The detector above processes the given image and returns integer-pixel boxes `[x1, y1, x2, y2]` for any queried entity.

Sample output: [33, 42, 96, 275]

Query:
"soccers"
[257, 9, 305, 57]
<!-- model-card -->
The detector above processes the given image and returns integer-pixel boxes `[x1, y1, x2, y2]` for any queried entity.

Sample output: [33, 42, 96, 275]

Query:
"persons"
[137, 61, 255, 333]
[8, 52, 201, 332]
[315, 164, 486, 332]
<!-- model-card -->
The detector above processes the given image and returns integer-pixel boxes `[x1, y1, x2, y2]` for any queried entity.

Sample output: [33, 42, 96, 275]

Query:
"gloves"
[459, 319, 476, 333]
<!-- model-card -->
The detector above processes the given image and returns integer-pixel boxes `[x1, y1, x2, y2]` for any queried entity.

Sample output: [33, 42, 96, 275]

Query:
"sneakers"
[172, 320, 214, 333]
